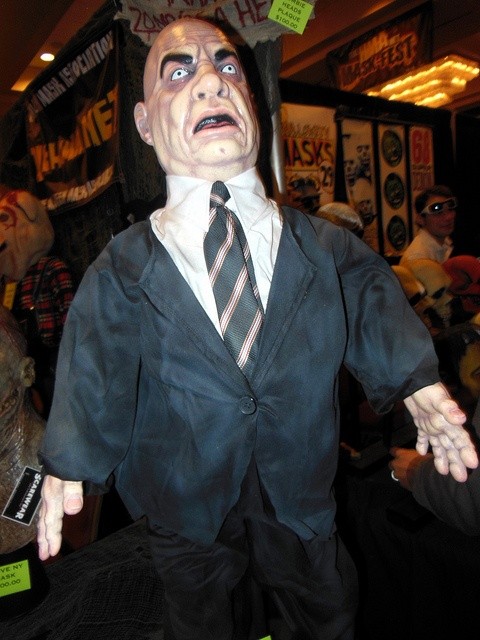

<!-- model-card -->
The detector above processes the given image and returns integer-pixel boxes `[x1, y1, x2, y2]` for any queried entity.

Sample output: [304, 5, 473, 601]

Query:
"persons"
[36, 17, 480, 634]
[398, 184, 458, 267]
[385, 444, 480, 538]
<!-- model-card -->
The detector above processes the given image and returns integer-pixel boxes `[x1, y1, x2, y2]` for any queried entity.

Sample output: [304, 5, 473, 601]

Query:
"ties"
[201, 179, 266, 387]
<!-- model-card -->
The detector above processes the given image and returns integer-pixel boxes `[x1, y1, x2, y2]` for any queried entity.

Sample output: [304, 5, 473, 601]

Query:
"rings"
[390, 470, 399, 482]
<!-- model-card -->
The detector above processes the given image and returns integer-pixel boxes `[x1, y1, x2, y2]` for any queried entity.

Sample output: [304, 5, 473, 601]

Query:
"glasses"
[418, 196, 458, 216]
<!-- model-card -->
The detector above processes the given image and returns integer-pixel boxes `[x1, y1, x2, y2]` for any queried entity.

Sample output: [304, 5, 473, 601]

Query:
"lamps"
[365, 40, 476, 127]
